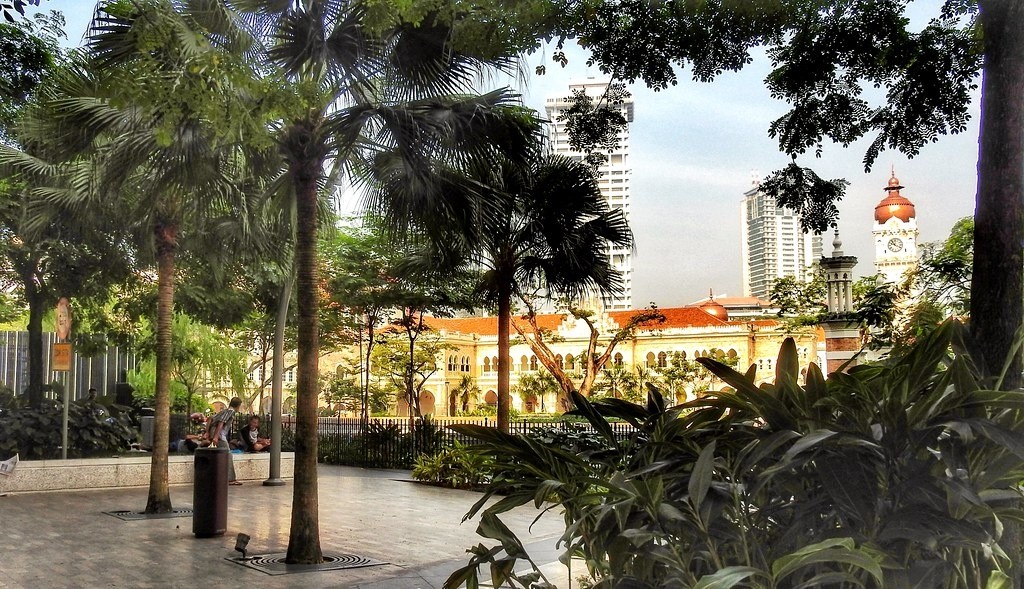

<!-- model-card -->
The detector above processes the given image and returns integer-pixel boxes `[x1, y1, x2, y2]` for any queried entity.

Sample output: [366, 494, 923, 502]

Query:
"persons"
[132, 397, 271, 486]
[87, 388, 97, 400]
[374, 415, 415, 432]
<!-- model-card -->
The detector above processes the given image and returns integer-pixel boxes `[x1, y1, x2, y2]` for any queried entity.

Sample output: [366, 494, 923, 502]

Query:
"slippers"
[228, 481, 243, 486]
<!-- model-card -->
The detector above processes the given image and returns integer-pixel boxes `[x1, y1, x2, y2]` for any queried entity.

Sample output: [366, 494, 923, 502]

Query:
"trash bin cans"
[140, 407, 155, 448]
[189, 445, 232, 538]
